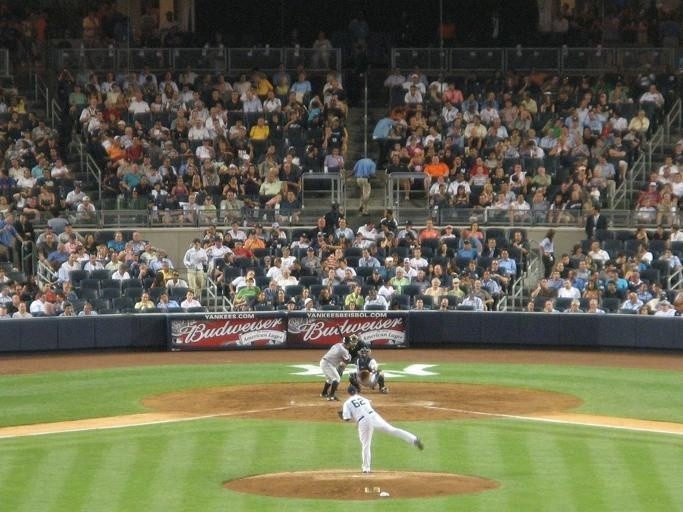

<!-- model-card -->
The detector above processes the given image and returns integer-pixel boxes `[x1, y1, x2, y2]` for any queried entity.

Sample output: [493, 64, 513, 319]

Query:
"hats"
[649, 182, 658, 188]
[343, 336, 353, 344]
[46, 224, 53, 230]
[82, 196, 91, 202]
[74, 180, 83, 188]
[244, 220, 471, 305]
[347, 385, 358, 395]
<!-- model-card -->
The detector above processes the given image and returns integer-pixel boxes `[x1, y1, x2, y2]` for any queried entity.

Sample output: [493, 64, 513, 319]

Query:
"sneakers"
[319, 391, 341, 401]
[379, 387, 389, 394]
[414, 436, 423, 450]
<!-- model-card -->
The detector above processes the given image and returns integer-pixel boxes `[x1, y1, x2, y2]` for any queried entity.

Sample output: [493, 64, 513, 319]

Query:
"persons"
[0, 1, 682, 318]
[319, 334, 390, 401]
[337, 385, 424, 473]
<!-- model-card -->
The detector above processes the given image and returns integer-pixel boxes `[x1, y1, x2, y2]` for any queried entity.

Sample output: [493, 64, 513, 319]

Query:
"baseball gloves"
[336, 410, 347, 420]
[358, 370, 370, 385]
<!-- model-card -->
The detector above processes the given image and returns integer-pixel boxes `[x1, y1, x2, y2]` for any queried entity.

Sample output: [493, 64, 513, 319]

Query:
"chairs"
[69, 115, 682, 317]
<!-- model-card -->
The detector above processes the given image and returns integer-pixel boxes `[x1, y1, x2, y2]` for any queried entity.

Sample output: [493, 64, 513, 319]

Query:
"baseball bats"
[337, 324, 343, 340]
